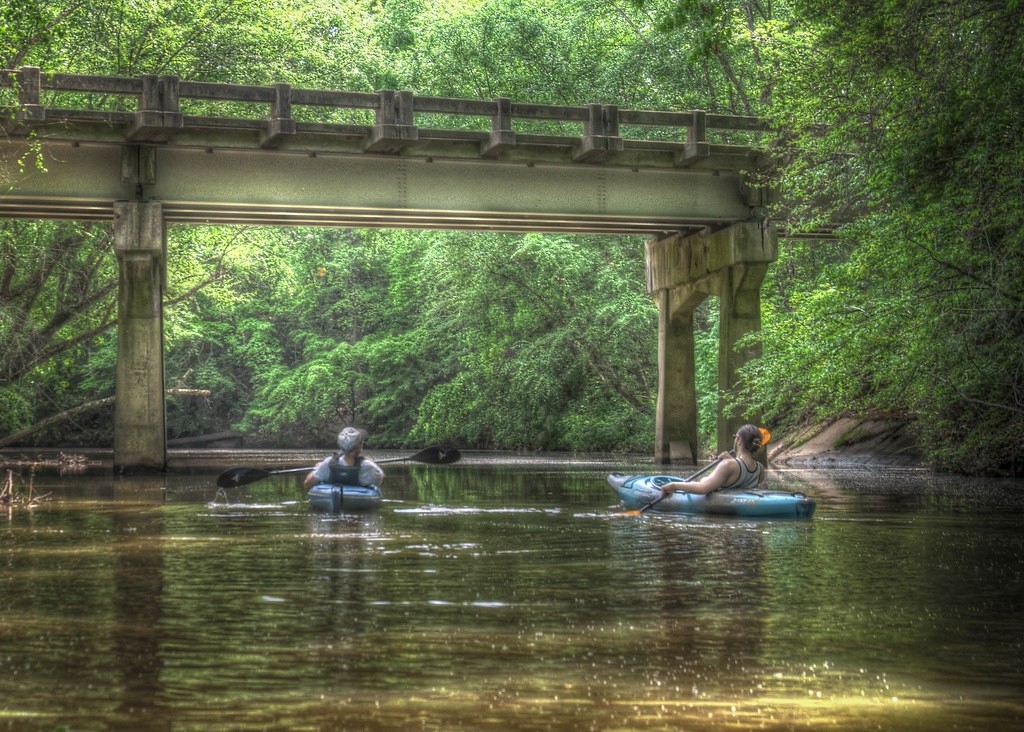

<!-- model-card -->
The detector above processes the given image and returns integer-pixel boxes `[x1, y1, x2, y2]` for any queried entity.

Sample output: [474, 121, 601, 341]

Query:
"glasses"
[732, 433, 739, 440]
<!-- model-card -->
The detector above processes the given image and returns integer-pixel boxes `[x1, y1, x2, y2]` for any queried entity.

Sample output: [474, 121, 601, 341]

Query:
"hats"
[337, 427, 368, 454]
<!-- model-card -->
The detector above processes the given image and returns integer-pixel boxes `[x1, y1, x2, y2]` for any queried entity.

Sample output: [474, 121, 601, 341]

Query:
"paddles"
[619, 448, 735, 517]
[215, 443, 462, 487]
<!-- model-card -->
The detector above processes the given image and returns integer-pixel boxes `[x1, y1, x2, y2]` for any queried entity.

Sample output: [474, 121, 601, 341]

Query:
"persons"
[303, 426, 387, 490]
[660, 423, 765, 494]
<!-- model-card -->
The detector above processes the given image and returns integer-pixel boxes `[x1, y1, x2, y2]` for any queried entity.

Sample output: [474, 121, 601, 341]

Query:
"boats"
[307, 483, 382, 512]
[606, 471, 816, 520]
